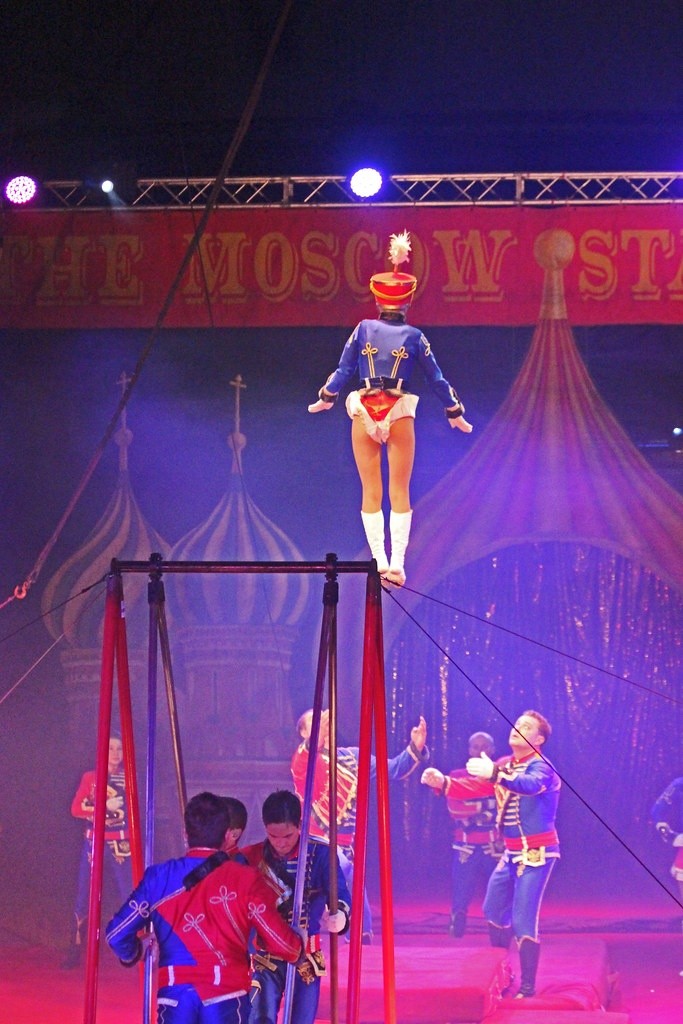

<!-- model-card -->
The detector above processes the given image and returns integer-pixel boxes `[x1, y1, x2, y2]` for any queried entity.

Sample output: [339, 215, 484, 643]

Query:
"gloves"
[420, 767, 452, 796]
[323, 909, 346, 933]
[139, 931, 159, 963]
[106, 796, 124, 811]
[448, 415, 473, 433]
[81, 797, 95, 811]
[308, 399, 334, 413]
[466, 751, 504, 783]
[291, 927, 308, 950]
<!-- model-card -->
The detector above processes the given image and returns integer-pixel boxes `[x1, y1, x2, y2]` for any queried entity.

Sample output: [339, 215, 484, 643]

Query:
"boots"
[361, 929, 374, 945]
[344, 936, 350, 945]
[487, 920, 514, 998]
[59, 912, 87, 971]
[449, 911, 466, 937]
[514, 935, 540, 999]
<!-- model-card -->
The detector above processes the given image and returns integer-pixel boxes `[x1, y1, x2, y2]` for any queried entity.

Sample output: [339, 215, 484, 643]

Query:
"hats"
[370, 272, 417, 310]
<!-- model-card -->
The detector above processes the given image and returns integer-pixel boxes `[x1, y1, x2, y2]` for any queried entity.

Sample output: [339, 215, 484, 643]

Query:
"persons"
[107, 790, 351, 1023]
[290, 706, 431, 946]
[420, 710, 561, 998]
[58, 728, 132, 971]
[650, 776, 683, 978]
[307, 273, 474, 587]
